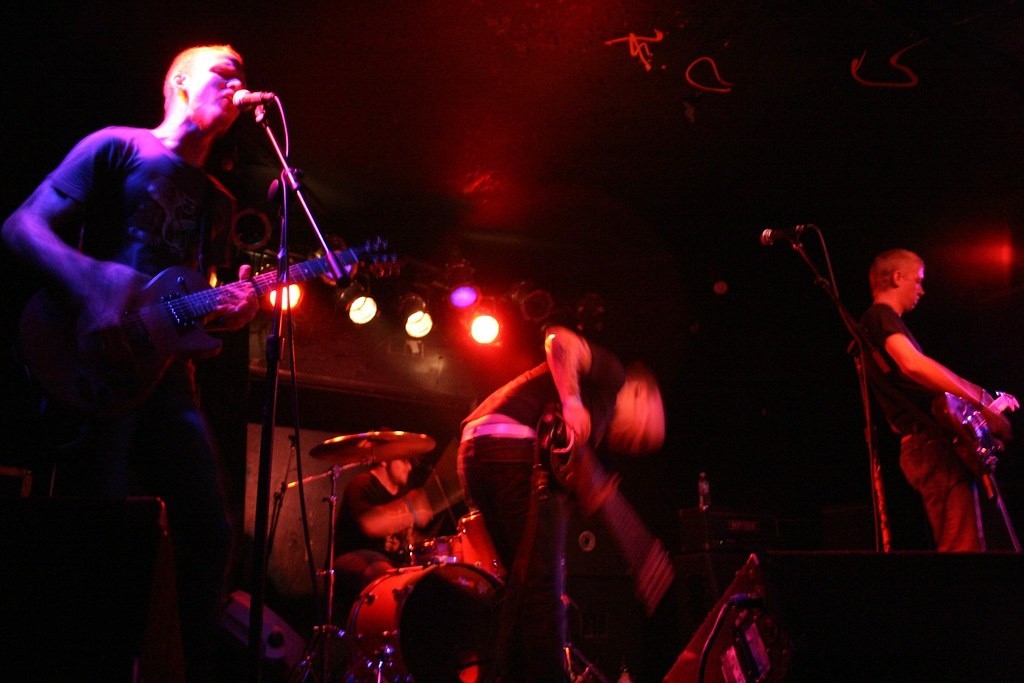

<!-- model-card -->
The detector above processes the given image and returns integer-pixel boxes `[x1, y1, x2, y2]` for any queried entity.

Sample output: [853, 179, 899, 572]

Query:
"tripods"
[288, 457, 380, 683]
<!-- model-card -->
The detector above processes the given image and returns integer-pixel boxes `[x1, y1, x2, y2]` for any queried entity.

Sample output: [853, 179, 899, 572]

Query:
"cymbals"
[308, 429, 437, 466]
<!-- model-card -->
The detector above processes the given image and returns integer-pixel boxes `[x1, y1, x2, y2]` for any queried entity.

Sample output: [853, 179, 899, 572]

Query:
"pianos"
[242, 366, 474, 436]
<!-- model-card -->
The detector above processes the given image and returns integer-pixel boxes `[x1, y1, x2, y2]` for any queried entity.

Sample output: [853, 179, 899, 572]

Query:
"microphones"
[761, 224, 815, 244]
[232, 90, 276, 110]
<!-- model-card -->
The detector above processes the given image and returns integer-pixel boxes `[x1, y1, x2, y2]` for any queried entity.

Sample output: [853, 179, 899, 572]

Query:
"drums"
[344, 560, 507, 683]
[217, 591, 311, 683]
[459, 509, 507, 580]
[320, 548, 395, 623]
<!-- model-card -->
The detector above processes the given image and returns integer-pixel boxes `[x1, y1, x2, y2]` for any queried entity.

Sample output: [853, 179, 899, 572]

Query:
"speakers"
[663, 547, 819, 683]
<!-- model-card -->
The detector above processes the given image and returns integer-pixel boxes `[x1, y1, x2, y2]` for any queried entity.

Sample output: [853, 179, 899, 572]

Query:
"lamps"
[446, 257, 501, 345]
[514, 278, 551, 323]
[398, 292, 433, 339]
[343, 282, 377, 325]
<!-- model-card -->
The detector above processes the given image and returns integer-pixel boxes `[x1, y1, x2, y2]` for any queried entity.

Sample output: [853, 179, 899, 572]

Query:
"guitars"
[19, 237, 412, 420]
[931, 389, 1021, 476]
[535, 406, 675, 619]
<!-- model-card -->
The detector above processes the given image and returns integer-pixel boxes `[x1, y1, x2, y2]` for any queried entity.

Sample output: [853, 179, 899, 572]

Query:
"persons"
[0, 44, 259, 646]
[858, 248, 1012, 553]
[456, 325, 666, 683]
[322, 459, 433, 603]
